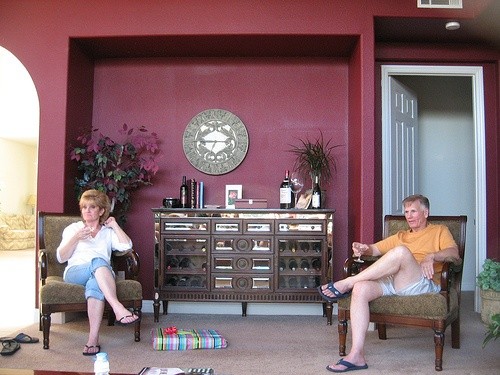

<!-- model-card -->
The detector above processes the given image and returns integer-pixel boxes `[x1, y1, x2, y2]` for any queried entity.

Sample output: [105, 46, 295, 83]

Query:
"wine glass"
[84, 224, 95, 240]
[287, 171, 306, 210]
[353, 242, 367, 263]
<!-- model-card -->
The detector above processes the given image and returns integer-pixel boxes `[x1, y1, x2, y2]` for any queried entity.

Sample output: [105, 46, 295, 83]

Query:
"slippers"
[0, 333, 39, 343]
[113, 312, 142, 326]
[317, 281, 351, 301]
[0, 340, 20, 355]
[326, 358, 368, 372]
[83, 344, 101, 355]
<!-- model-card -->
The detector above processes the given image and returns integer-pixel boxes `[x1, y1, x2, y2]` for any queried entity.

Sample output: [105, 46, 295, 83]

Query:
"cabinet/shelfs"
[152, 209, 337, 327]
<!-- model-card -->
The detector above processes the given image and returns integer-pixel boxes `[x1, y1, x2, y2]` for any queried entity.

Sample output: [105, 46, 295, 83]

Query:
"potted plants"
[66, 119, 159, 320]
[475, 258, 500, 329]
[291, 127, 345, 209]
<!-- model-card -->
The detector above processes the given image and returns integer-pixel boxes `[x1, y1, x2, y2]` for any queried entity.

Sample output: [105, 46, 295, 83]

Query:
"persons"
[316, 194, 459, 373]
[56, 190, 141, 356]
[229, 192, 238, 205]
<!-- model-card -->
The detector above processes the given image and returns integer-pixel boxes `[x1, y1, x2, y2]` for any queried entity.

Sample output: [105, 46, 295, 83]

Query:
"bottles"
[180, 175, 189, 208]
[93, 353, 111, 375]
[312, 175, 322, 209]
[279, 170, 296, 209]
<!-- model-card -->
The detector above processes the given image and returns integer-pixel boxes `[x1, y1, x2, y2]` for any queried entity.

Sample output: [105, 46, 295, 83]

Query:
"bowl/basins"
[162, 197, 179, 207]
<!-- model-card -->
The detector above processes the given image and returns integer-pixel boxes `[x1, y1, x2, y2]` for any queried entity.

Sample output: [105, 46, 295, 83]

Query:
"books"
[187, 179, 203, 208]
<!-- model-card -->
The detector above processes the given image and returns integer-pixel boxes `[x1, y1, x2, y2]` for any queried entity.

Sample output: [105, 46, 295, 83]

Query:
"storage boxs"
[235, 199, 267, 208]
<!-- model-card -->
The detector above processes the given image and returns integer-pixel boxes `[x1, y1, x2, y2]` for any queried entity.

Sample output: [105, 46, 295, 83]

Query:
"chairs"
[337, 216, 466, 371]
[36, 210, 143, 349]
